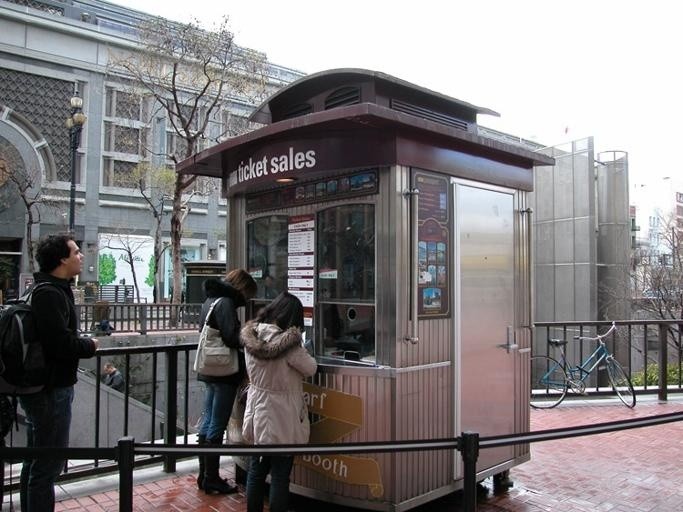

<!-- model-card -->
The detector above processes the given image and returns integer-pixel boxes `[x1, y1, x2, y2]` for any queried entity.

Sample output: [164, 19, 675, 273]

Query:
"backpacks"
[0, 283, 69, 396]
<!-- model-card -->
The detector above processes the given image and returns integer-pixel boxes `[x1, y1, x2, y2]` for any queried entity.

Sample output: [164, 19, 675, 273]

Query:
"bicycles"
[530, 322, 638, 409]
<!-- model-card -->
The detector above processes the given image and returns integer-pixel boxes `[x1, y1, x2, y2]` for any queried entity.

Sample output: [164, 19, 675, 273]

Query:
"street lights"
[66, 90, 88, 288]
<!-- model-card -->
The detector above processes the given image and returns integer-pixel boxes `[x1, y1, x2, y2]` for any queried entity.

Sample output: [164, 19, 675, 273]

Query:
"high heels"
[198, 472, 238, 495]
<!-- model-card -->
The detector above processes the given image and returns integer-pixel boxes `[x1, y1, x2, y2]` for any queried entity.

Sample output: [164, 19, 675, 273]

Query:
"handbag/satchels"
[194, 324, 241, 378]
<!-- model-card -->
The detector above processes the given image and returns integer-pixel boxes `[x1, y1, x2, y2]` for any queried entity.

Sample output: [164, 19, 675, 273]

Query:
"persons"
[19, 232, 99, 512]
[104, 364, 124, 393]
[239, 291, 317, 512]
[197, 269, 257, 494]
